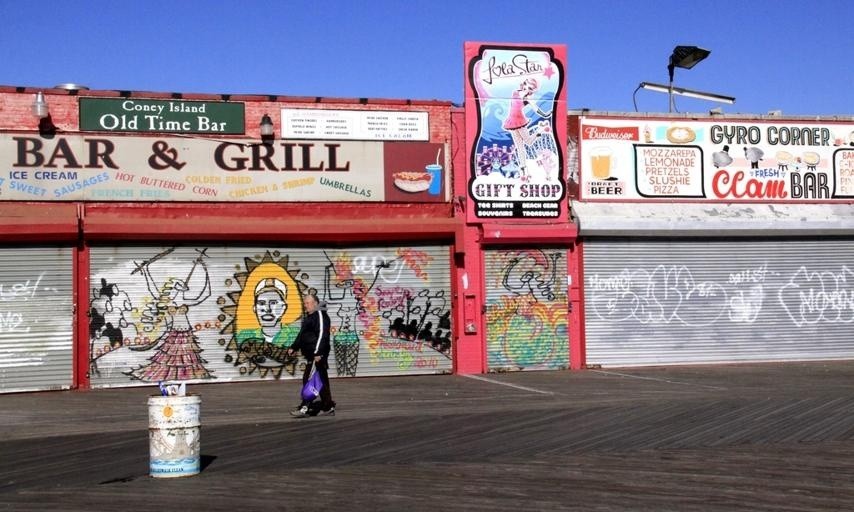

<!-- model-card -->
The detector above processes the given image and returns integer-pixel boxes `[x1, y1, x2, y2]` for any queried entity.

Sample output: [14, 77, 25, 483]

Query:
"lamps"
[632, 82, 735, 112]
[667, 45, 711, 113]
[32, 92, 48, 118]
[259, 113, 274, 136]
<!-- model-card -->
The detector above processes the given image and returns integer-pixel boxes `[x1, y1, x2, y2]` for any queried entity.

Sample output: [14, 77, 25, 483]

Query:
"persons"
[235, 277, 303, 346]
[287, 293, 337, 417]
[503, 78, 553, 177]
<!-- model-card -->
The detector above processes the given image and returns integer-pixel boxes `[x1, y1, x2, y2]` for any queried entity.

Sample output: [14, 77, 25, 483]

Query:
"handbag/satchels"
[301, 369, 324, 401]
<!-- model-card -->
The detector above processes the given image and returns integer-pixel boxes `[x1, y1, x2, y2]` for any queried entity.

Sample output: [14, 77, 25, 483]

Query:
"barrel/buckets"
[147, 395, 200, 479]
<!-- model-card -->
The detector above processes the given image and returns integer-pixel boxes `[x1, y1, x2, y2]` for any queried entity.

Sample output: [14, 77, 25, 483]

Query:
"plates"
[394, 173, 432, 193]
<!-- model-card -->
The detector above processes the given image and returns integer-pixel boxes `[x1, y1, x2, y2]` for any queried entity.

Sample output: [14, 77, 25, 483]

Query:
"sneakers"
[290, 410, 310, 418]
[315, 406, 335, 416]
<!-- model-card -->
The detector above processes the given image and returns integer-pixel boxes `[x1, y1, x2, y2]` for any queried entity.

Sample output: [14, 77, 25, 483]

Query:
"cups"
[589, 147, 612, 180]
[425, 164, 443, 196]
